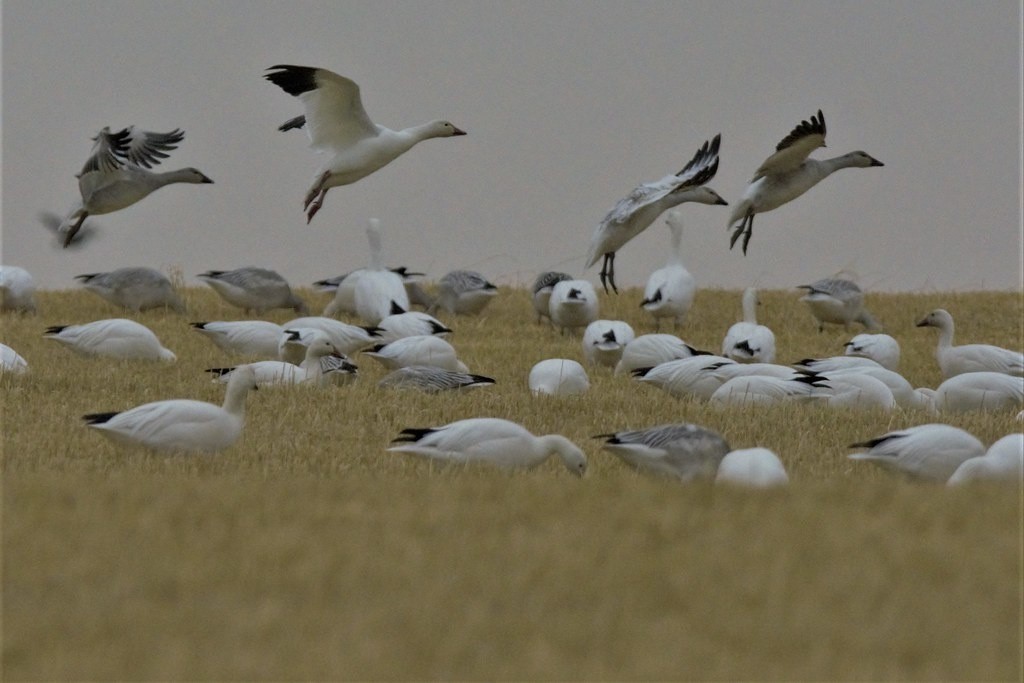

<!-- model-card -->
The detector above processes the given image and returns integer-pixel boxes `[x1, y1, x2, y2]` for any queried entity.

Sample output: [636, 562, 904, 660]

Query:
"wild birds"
[585, 132, 728, 295]
[62, 125, 215, 248]
[730, 108, 886, 257]
[1, 210, 1024, 493]
[262, 64, 468, 224]
